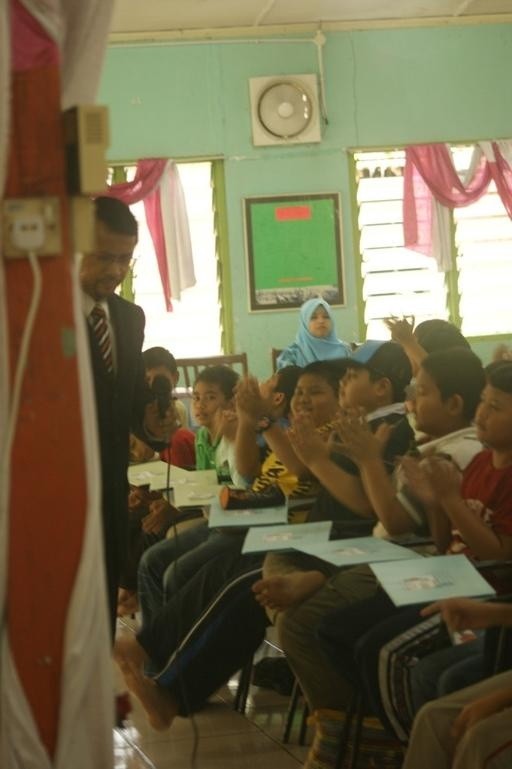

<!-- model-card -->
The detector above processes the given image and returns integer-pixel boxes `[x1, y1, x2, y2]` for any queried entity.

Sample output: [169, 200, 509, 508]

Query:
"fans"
[249, 73, 323, 147]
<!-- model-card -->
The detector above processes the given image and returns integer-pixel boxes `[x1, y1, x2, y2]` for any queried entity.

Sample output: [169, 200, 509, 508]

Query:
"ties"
[91, 304, 113, 375]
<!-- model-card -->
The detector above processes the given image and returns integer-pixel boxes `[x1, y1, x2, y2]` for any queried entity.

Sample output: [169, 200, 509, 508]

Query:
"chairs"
[128, 341, 511, 768]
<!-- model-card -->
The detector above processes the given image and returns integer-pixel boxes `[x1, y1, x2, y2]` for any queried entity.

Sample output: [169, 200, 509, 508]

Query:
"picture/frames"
[242, 191, 347, 314]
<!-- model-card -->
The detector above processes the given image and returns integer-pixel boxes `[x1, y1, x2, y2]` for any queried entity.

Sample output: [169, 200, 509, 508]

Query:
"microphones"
[152, 374, 171, 418]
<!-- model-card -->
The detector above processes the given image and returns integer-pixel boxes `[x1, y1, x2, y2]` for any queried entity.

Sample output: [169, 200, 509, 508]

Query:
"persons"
[75, 191, 183, 672]
[117, 294, 511, 768]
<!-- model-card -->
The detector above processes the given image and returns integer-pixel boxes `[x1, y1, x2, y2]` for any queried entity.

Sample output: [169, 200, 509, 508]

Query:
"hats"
[335, 340, 413, 389]
